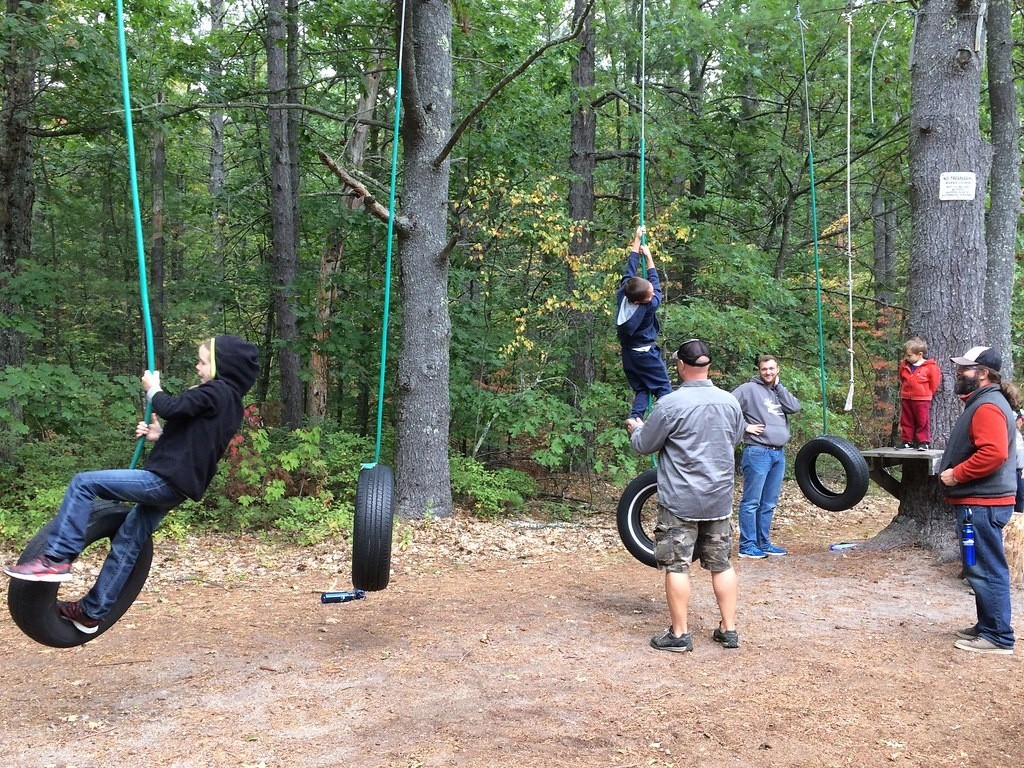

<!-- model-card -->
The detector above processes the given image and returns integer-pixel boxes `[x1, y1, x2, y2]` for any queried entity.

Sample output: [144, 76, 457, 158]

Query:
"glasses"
[959, 365, 983, 372]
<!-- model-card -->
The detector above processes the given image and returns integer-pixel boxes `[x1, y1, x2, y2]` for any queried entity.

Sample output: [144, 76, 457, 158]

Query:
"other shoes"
[624, 418, 633, 439]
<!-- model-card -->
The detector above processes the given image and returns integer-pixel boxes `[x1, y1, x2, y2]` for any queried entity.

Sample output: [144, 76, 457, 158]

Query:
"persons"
[938, 347, 1024, 654]
[727, 355, 802, 559]
[896, 337, 941, 450]
[627, 339, 744, 651]
[4, 334, 263, 634]
[616, 223, 674, 438]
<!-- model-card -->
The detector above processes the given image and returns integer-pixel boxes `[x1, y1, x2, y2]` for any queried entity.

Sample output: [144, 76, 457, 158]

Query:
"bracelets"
[952, 475, 958, 483]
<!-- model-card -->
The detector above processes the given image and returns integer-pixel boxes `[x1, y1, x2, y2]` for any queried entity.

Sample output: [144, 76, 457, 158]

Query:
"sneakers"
[738, 545, 768, 559]
[956, 627, 980, 639]
[894, 441, 914, 450]
[56, 599, 99, 634]
[649, 624, 693, 652]
[713, 620, 739, 648]
[3, 553, 73, 582]
[917, 442, 928, 451]
[757, 544, 788, 556]
[954, 637, 1014, 654]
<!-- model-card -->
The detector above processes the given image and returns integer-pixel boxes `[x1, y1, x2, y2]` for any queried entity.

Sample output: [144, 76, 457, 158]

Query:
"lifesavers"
[9, 506, 153, 649]
[352, 465, 394, 592]
[795, 435, 870, 513]
[616, 467, 698, 567]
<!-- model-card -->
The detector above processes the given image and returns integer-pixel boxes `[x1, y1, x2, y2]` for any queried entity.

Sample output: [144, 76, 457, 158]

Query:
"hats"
[1012, 409, 1024, 421]
[672, 338, 712, 367]
[949, 346, 1002, 372]
